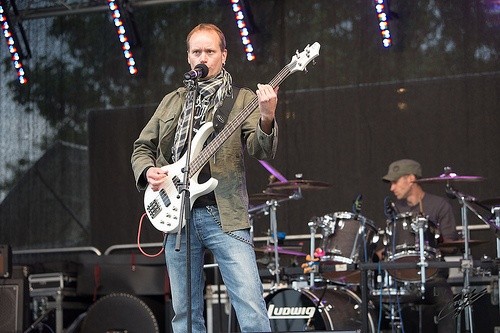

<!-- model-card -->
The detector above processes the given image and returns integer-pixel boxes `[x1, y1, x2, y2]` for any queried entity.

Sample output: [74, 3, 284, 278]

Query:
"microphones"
[356, 195, 363, 212]
[448, 189, 479, 203]
[386, 196, 400, 216]
[183, 64, 209, 80]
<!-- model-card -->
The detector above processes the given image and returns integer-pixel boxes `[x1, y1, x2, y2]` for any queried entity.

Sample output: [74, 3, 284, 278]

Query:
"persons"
[131, 23, 279, 333]
[372, 159, 459, 333]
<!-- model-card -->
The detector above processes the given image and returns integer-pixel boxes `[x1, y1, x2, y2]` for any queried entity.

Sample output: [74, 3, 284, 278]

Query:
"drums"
[312, 210, 379, 287]
[263, 285, 379, 333]
[369, 266, 422, 304]
[382, 211, 445, 284]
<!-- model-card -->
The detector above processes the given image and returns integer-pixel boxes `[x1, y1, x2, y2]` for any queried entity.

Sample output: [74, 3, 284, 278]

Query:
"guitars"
[142, 42, 321, 235]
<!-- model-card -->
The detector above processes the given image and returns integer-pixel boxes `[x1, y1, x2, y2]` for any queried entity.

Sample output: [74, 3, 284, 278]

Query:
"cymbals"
[438, 239, 492, 247]
[260, 179, 334, 197]
[409, 174, 492, 183]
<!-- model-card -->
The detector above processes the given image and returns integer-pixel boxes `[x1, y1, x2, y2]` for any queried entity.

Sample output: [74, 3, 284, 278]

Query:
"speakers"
[0, 277, 28, 333]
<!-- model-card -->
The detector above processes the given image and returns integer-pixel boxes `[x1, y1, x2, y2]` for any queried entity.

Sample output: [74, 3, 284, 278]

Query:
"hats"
[381, 159, 422, 184]
